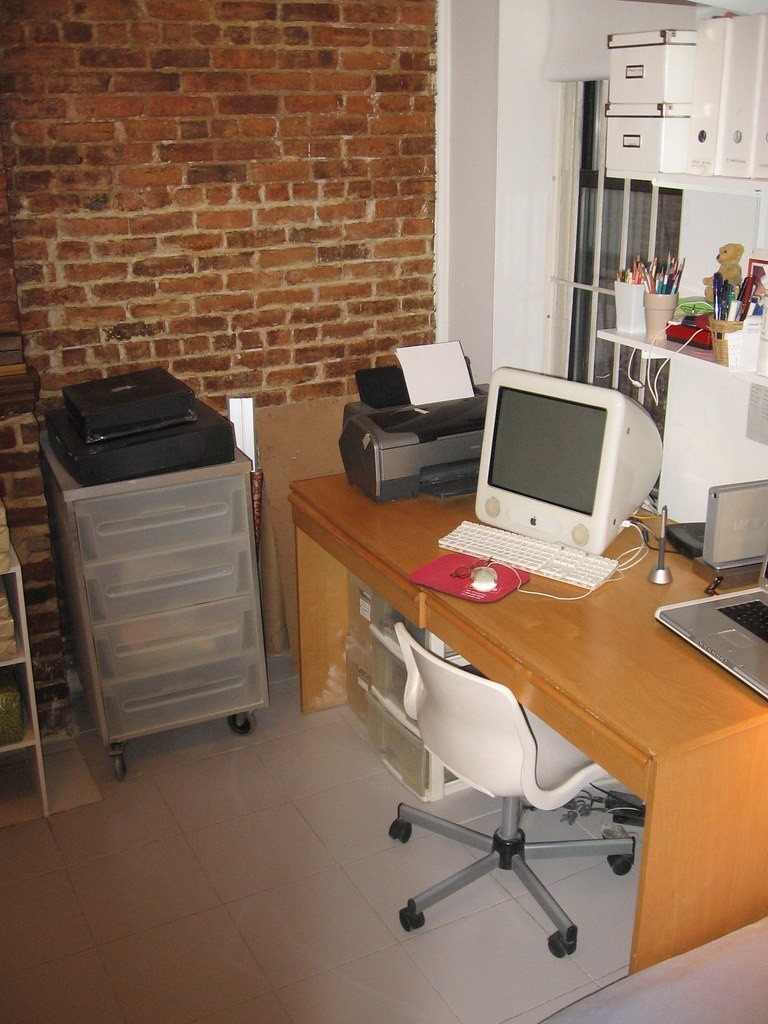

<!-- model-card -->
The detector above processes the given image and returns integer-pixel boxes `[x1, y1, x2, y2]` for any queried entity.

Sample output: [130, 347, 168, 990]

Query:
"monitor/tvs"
[475, 367, 664, 555]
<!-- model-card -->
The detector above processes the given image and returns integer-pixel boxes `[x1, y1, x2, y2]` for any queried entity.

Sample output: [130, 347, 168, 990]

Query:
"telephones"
[665, 312, 714, 351]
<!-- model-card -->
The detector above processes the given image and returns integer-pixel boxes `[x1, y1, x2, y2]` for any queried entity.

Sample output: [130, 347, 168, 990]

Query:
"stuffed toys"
[703, 243, 744, 300]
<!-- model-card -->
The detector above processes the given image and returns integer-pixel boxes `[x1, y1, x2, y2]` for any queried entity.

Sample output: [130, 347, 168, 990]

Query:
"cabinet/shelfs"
[39, 423, 270, 778]
[0, 539, 50, 820]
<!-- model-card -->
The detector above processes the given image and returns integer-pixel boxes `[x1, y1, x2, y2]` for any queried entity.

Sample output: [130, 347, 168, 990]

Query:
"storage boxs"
[345, 569, 488, 803]
[665, 322, 713, 350]
[603, 30, 697, 175]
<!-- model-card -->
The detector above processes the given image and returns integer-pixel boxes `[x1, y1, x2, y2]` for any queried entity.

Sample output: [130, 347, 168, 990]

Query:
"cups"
[615, 280, 647, 334]
[644, 291, 679, 339]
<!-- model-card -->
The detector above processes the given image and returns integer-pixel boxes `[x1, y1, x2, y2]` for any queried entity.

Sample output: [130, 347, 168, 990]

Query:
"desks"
[288, 473, 768, 975]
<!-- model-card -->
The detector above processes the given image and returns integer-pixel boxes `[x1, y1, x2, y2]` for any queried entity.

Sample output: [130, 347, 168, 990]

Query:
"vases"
[614, 279, 680, 341]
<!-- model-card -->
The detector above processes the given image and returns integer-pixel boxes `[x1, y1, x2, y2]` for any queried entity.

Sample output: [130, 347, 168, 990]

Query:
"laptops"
[654, 548, 768, 704]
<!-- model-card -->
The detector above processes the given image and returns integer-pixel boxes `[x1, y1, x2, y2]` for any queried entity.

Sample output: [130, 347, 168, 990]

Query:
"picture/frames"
[748, 258, 768, 307]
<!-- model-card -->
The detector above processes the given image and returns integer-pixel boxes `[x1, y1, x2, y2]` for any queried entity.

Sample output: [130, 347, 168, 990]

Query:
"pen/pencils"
[616, 250, 686, 295]
[712, 271, 765, 322]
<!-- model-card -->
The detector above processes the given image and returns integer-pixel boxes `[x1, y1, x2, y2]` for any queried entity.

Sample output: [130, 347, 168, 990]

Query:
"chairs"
[389, 622, 637, 960]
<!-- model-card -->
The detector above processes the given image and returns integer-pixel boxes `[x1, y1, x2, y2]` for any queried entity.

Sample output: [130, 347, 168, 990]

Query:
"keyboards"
[438, 522, 618, 590]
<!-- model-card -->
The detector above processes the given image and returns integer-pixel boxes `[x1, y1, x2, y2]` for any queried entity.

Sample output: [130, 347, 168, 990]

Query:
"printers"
[339, 354, 491, 504]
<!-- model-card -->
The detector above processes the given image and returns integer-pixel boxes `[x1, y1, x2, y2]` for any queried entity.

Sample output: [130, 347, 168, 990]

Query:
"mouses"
[471, 566, 498, 593]
